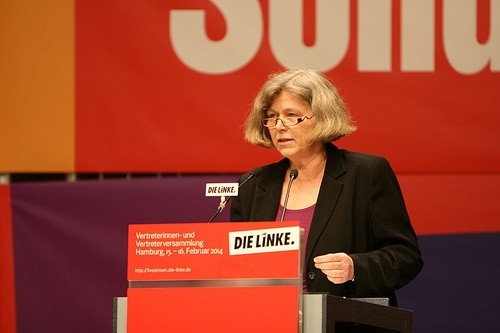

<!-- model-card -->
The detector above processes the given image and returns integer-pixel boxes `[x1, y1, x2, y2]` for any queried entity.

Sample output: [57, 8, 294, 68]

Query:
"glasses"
[261, 115, 313, 127]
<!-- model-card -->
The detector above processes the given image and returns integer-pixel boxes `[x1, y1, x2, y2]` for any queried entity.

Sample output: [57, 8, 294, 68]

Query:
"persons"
[229, 68, 425, 308]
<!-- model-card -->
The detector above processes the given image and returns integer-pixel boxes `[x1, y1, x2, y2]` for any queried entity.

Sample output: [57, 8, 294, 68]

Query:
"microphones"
[280, 169, 298, 222]
[208, 168, 261, 222]
